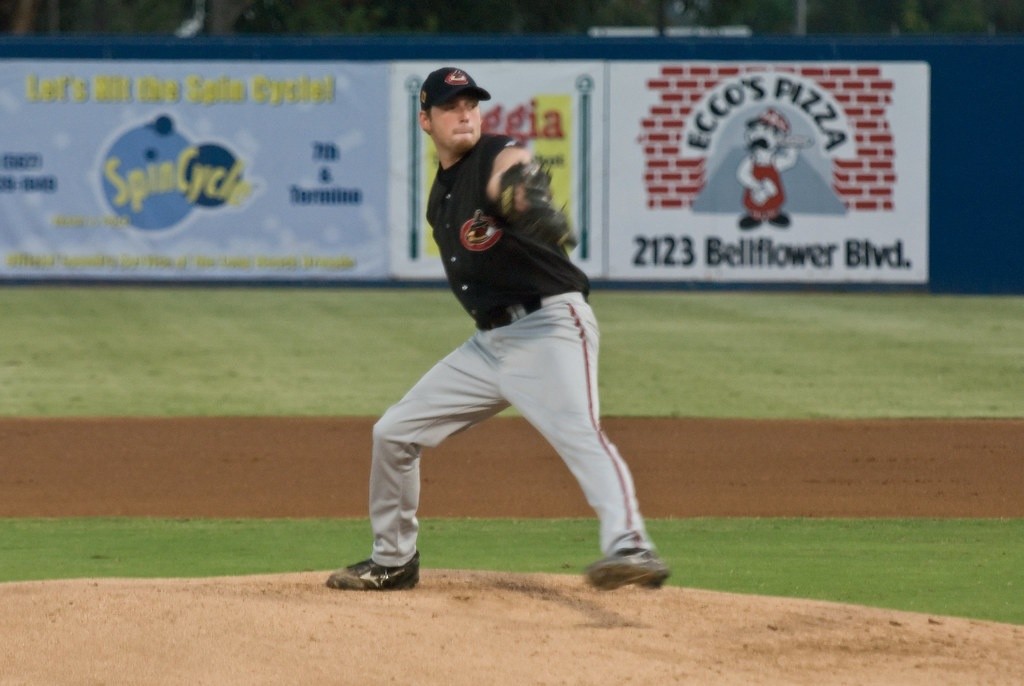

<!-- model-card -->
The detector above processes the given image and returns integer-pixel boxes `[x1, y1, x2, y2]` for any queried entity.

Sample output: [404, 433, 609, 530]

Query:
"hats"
[420, 68, 492, 109]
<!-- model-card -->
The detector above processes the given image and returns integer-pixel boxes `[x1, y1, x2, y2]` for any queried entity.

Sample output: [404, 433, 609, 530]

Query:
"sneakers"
[326, 552, 420, 590]
[590, 548, 670, 591]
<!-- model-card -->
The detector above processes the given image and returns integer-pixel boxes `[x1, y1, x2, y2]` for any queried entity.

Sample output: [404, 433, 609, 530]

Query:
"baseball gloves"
[494, 162, 580, 254]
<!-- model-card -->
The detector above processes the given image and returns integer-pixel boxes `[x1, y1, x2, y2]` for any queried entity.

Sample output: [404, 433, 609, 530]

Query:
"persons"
[325, 68, 671, 593]
